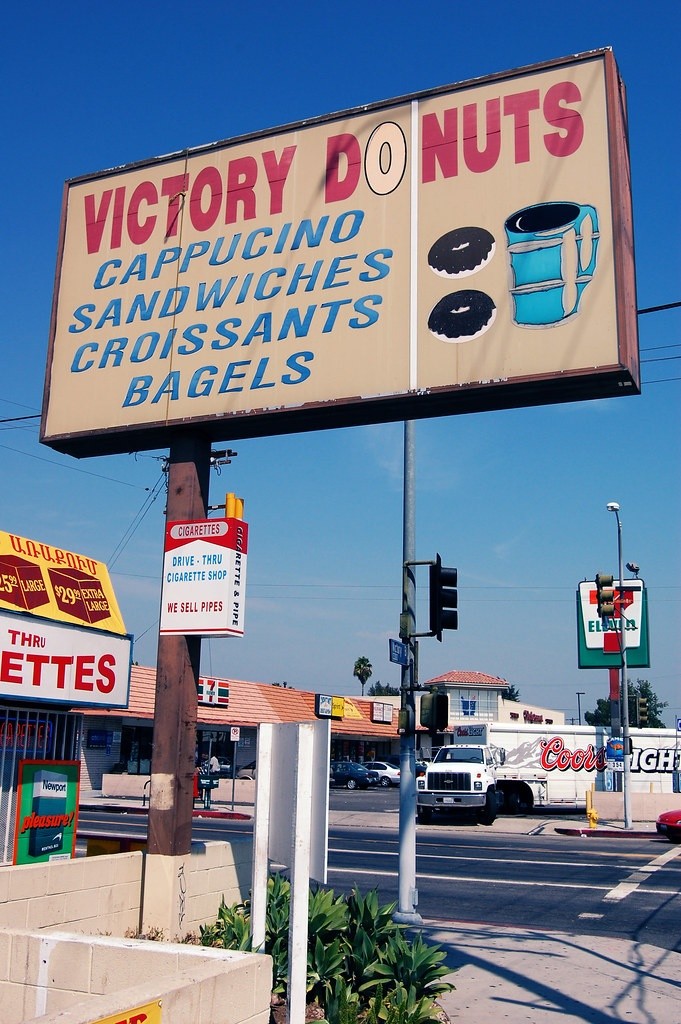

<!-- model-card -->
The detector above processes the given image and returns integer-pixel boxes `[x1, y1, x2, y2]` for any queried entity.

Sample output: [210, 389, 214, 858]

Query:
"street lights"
[576, 691, 585, 724]
[604, 501, 634, 829]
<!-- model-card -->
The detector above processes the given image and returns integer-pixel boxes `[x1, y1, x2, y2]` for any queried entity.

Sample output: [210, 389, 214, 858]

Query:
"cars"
[238, 759, 257, 781]
[216, 756, 232, 776]
[357, 761, 401, 787]
[329, 761, 379, 790]
[655, 808, 681, 843]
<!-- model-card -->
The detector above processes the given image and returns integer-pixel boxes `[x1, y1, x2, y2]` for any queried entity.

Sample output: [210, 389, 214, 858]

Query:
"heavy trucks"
[414, 722, 681, 826]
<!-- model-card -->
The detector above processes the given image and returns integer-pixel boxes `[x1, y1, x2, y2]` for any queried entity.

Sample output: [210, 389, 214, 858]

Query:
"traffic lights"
[634, 690, 648, 726]
[419, 690, 450, 730]
[428, 552, 459, 643]
[595, 572, 616, 618]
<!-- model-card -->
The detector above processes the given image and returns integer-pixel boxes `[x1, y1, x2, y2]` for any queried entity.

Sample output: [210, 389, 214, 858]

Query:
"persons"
[210, 753, 220, 775]
